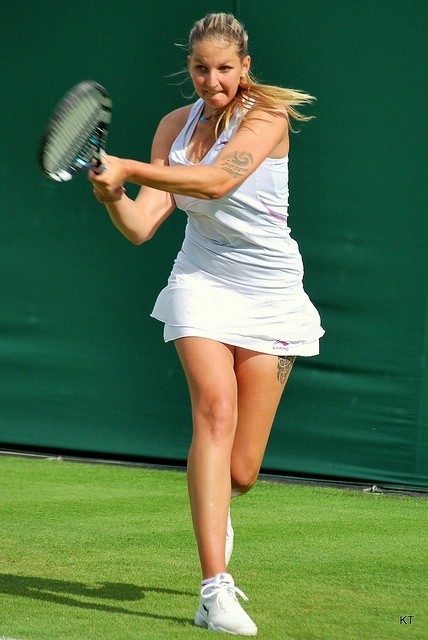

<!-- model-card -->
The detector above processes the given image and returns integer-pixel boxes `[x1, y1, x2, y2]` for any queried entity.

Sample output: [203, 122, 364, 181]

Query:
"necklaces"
[199, 111, 224, 122]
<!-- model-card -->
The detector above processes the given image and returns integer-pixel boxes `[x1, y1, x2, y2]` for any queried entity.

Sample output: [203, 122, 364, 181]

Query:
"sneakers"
[193, 573, 256, 637]
[225, 505, 234, 569]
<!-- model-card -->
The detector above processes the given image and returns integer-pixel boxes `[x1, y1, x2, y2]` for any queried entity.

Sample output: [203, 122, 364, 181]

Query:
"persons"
[88, 12, 327, 636]
